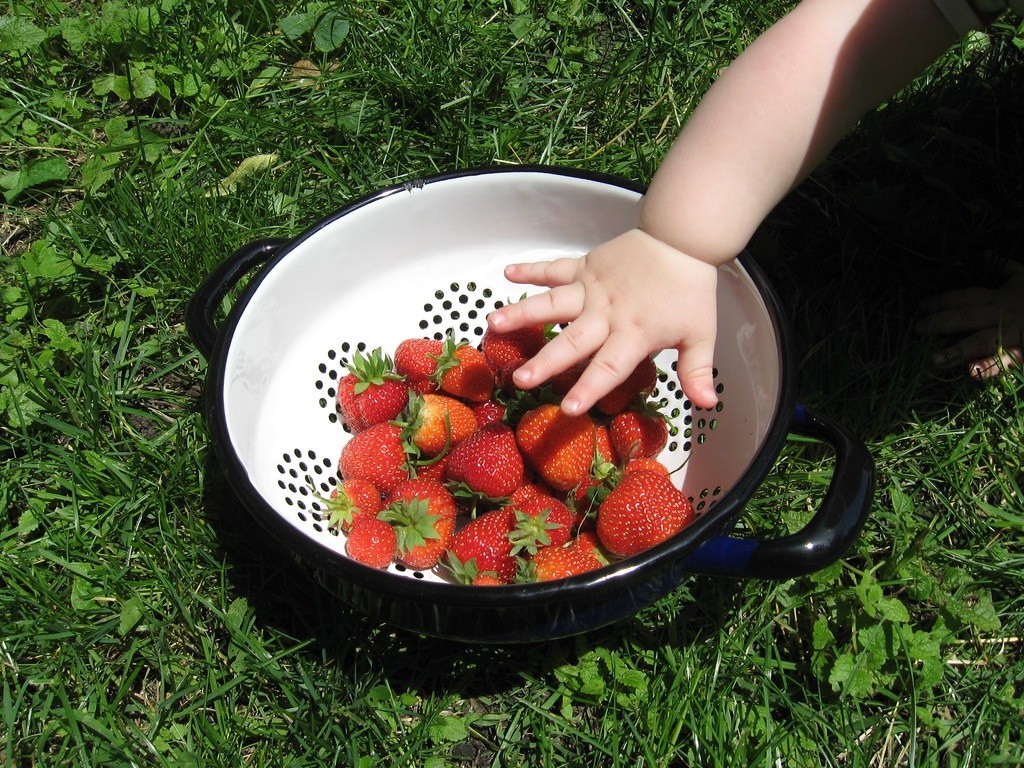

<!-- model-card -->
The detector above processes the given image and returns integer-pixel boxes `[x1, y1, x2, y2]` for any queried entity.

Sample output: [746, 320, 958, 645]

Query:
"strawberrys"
[325, 293, 695, 585]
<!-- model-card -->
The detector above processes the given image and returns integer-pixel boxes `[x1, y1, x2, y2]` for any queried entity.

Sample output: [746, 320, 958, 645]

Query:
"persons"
[490, 0, 1024, 418]
